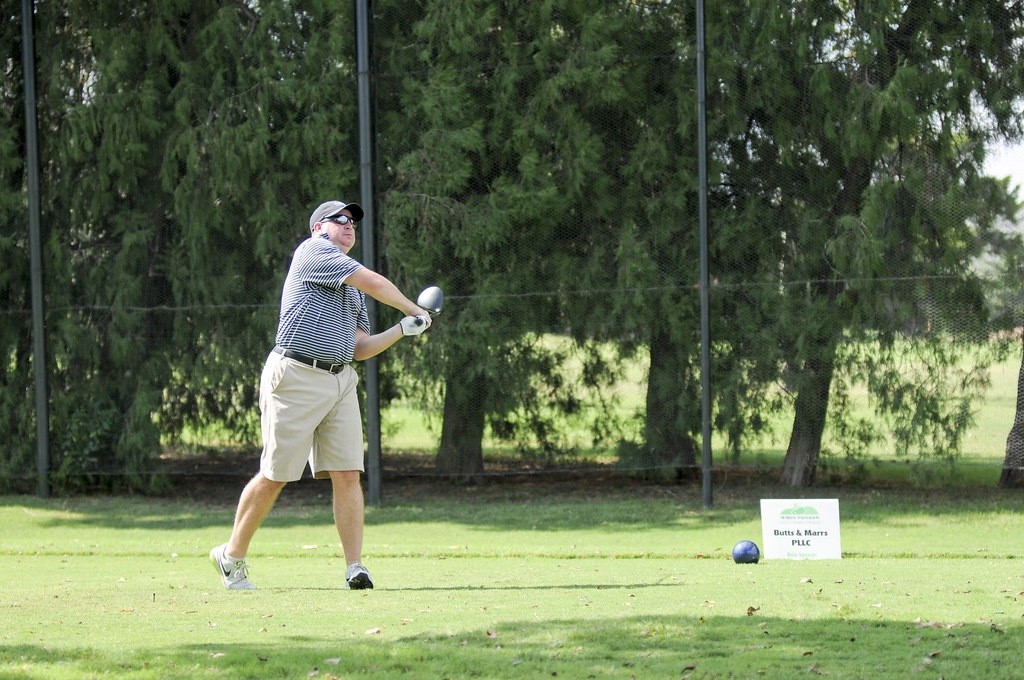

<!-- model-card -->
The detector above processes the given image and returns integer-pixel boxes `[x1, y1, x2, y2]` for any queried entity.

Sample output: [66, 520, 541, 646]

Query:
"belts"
[274, 346, 345, 375]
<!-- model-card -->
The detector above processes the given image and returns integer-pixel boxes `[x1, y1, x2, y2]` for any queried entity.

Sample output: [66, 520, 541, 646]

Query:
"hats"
[310, 201, 364, 233]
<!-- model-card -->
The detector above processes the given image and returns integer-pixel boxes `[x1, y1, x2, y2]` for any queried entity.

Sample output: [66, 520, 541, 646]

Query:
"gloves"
[399, 314, 431, 336]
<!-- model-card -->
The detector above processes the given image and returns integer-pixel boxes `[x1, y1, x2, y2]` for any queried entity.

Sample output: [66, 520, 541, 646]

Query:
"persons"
[210, 201, 432, 589]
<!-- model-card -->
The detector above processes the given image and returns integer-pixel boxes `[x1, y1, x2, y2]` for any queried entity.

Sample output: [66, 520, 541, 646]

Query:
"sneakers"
[211, 549, 252, 591]
[347, 567, 373, 590]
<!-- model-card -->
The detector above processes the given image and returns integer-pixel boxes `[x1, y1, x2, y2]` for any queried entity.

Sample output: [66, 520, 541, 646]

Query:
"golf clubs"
[412, 286, 444, 326]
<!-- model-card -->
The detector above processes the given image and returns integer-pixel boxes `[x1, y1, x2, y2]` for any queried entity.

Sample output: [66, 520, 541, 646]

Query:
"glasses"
[316, 214, 358, 229]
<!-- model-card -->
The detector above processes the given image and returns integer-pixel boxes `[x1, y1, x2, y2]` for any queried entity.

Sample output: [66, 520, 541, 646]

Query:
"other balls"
[732, 540, 760, 564]
